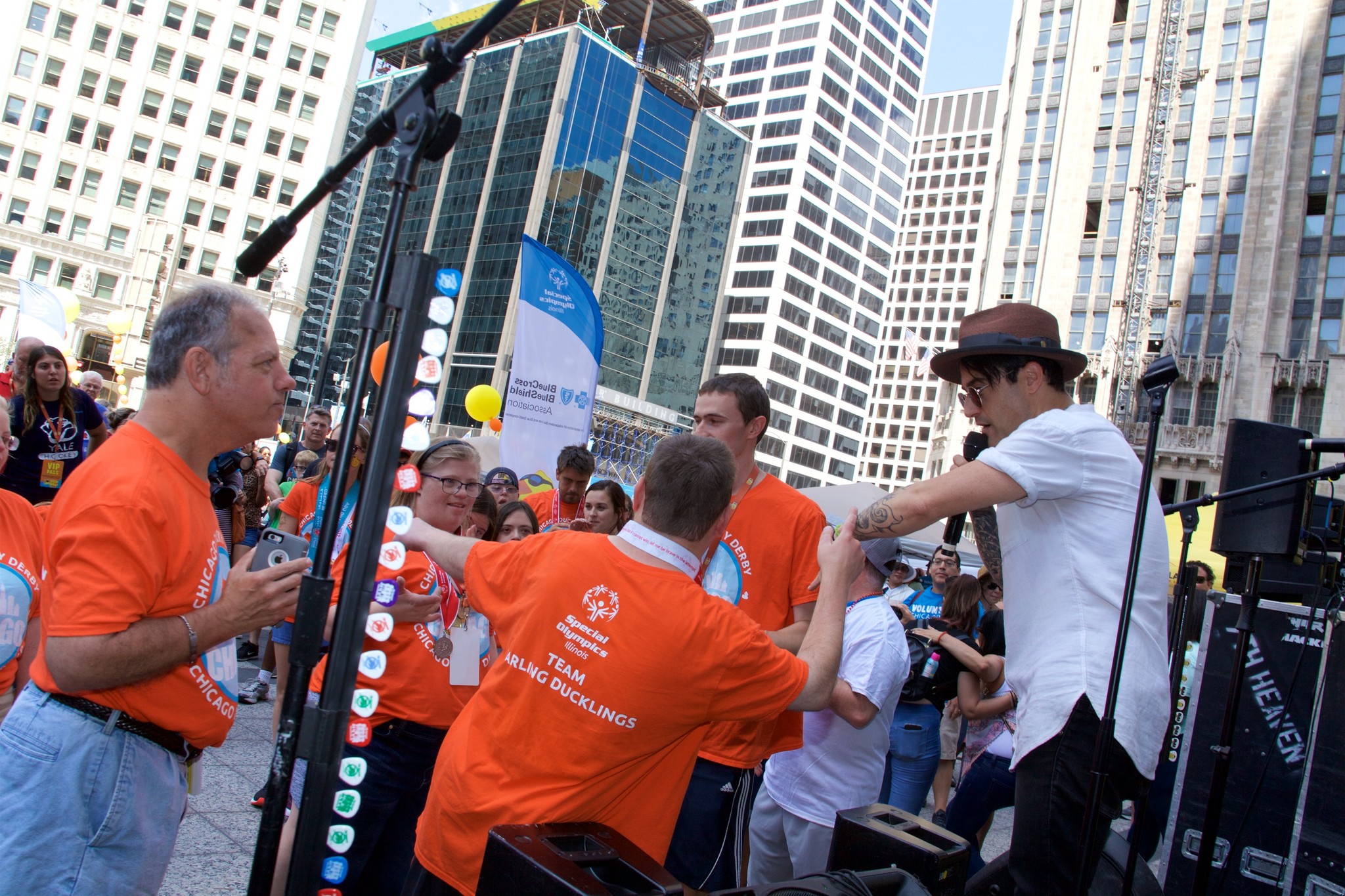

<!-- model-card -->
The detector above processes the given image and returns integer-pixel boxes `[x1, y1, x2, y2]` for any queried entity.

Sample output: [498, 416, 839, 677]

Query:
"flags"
[494, 232, 603, 487]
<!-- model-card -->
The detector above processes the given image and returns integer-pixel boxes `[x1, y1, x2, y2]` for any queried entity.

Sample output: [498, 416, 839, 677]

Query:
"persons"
[663, 372, 829, 894]
[1169, 561, 1216, 646]
[206, 405, 631, 896]
[0, 283, 312, 896]
[748, 535, 1016, 886]
[806, 303, 1172, 896]
[0, 337, 136, 723]
[392, 435, 864, 896]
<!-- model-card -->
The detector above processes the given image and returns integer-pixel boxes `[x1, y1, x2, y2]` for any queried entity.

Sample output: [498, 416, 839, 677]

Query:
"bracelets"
[1010, 691, 1016, 709]
[834, 523, 842, 536]
[937, 631, 948, 644]
[1012, 690, 1018, 705]
[177, 614, 198, 664]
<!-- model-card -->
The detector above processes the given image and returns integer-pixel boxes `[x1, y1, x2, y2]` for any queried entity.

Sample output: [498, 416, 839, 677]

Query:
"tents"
[790, 481, 986, 571]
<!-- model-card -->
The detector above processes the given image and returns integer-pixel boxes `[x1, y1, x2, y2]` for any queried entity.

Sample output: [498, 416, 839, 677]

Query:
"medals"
[434, 632, 453, 658]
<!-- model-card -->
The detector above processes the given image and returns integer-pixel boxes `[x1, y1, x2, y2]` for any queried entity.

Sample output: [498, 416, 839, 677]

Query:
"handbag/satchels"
[232, 503, 246, 544]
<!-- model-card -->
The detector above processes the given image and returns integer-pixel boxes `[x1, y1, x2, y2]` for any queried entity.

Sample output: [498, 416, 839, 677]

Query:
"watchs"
[255, 456, 265, 462]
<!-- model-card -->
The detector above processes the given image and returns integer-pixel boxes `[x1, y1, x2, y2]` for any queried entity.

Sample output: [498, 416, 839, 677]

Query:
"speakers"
[474, 821, 684, 896]
[824, 803, 970, 896]
[1211, 417, 1313, 556]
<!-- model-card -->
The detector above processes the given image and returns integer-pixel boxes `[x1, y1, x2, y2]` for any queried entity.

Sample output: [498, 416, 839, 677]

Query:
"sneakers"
[236, 640, 260, 662]
[237, 676, 270, 704]
[251, 781, 269, 805]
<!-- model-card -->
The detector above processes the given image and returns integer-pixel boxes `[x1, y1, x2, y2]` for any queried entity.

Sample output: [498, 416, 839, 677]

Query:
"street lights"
[331, 353, 358, 432]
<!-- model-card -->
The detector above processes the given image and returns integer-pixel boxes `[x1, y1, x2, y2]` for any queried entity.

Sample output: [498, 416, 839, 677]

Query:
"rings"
[959, 710, 960, 711]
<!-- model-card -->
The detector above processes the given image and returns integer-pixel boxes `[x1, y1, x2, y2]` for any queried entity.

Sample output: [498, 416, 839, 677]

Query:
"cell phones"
[250, 527, 309, 592]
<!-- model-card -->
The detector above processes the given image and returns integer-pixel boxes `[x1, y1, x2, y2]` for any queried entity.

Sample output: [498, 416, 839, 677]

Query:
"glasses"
[933, 557, 959, 568]
[325, 438, 367, 456]
[894, 565, 907, 572]
[263, 452, 271, 456]
[397, 461, 406, 468]
[1195, 575, 1208, 585]
[80, 383, 102, 392]
[490, 486, 518, 494]
[415, 473, 487, 499]
[295, 465, 307, 472]
[987, 582, 1003, 591]
[958, 367, 1025, 409]
[1, 434, 19, 451]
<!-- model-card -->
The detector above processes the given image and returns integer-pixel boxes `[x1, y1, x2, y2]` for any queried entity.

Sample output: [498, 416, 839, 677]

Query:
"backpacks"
[898, 617, 972, 702]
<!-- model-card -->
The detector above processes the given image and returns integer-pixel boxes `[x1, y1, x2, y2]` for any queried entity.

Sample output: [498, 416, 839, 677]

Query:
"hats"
[887, 555, 917, 583]
[859, 536, 902, 578]
[930, 304, 1087, 388]
[485, 468, 519, 490]
[400, 448, 412, 457]
[978, 566, 990, 580]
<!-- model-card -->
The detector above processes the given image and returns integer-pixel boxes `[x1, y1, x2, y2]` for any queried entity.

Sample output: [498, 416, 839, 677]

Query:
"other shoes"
[1120, 806, 1132, 820]
[931, 809, 947, 828]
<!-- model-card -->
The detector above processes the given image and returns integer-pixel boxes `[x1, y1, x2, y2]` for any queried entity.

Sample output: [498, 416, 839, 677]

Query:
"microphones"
[1298, 436, 1345, 453]
[942, 432, 988, 554]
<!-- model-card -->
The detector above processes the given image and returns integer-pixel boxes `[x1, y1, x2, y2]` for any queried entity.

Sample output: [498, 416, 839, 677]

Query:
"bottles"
[922, 652, 940, 679]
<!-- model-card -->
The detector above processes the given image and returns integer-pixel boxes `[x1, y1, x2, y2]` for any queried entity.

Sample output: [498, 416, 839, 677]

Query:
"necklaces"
[846, 591, 883, 615]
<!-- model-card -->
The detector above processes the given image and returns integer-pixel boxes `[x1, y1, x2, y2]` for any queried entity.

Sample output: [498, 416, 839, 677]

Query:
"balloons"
[279, 432, 290, 444]
[370, 339, 423, 389]
[465, 384, 502, 433]
[404, 416, 418, 429]
[275, 423, 282, 434]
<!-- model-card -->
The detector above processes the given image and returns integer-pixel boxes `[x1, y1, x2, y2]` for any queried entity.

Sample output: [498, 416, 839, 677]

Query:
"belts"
[34, 684, 201, 765]
